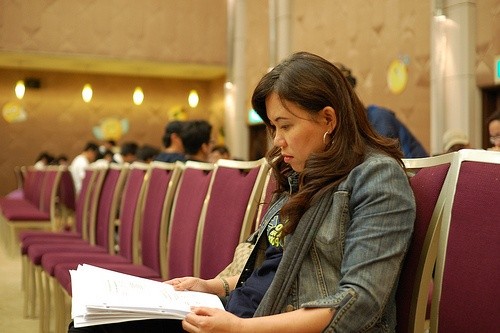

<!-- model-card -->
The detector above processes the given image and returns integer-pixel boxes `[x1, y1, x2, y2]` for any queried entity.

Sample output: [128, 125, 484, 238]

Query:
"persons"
[66, 52, 416, 333]
[32, 62, 500, 201]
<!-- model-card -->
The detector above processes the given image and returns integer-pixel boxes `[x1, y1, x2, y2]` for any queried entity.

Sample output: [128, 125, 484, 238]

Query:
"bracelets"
[219, 276, 230, 298]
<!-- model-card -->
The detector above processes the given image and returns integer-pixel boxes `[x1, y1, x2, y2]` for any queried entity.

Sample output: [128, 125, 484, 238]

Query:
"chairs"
[0, 148, 500, 333]
